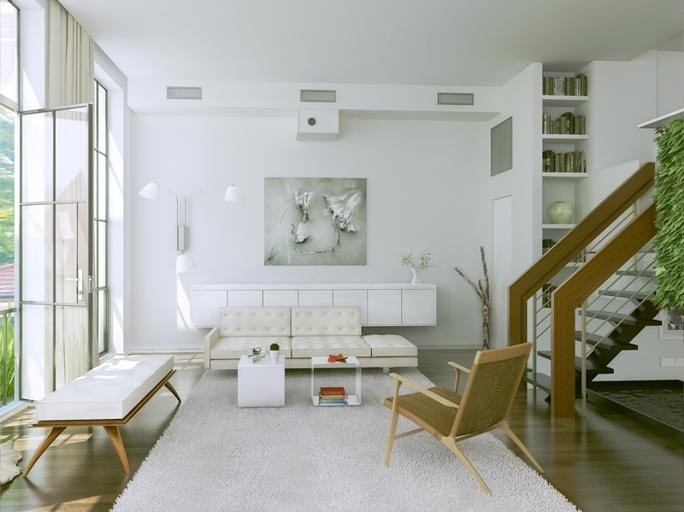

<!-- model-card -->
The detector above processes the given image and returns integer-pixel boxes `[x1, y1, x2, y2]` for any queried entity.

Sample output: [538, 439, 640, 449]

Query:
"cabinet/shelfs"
[369, 289, 402, 327]
[402, 289, 436, 328]
[334, 289, 368, 326]
[298, 290, 334, 306]
[540, 90, 589, 312]
[262, 290, 297, 307]
[228, 290, 262, 308]
[191, 289, 228, 329]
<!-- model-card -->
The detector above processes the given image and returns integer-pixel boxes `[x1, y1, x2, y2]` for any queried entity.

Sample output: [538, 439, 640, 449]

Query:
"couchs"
[202, 307, 420, 376]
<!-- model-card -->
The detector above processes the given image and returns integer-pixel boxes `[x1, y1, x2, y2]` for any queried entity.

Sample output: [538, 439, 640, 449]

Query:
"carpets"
[104, 364, 587, 512]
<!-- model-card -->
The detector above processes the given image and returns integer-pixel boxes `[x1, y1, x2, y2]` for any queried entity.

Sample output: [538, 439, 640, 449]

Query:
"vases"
[546, 201, 577, 224]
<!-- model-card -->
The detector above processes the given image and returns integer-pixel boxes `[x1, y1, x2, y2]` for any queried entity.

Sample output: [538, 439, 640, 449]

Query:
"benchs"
[15, 353, 183, 481]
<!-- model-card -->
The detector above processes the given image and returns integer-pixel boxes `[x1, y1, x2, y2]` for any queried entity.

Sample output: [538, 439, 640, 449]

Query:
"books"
[542, 72, 588, 173]
[318, 386, 348, 407]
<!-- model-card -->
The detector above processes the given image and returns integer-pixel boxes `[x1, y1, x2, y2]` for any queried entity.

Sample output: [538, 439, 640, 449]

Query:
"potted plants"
[268, 342, 280, 360]
[398, 247, 433, 285]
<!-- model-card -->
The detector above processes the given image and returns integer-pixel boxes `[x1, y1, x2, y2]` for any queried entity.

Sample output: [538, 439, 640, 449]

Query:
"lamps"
[135, 180, 243, 255]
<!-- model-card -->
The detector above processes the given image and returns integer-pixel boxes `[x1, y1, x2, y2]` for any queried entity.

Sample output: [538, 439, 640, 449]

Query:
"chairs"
[380, 342, 547, 496]
